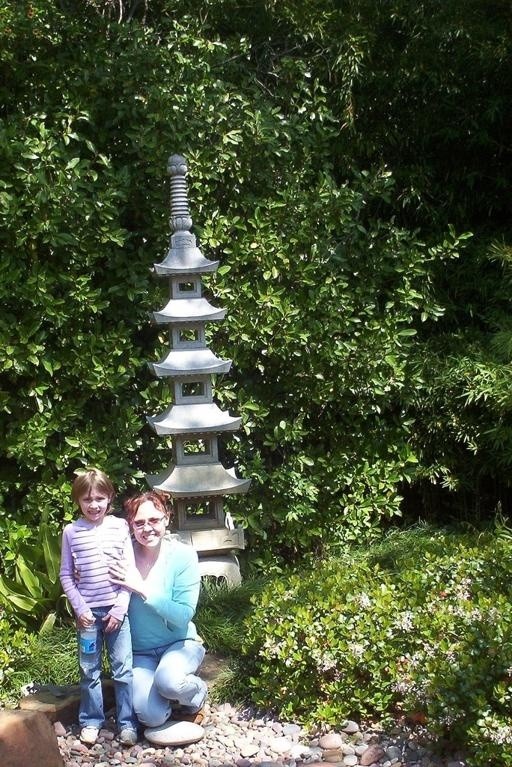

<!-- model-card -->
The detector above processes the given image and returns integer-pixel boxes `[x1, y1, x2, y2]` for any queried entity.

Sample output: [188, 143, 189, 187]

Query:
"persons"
[101, 489, 209, 731]
[57, 466, 141, 748]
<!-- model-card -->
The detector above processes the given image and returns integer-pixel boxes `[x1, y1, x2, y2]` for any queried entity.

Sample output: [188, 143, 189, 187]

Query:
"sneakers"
[177, 700, 205, 723]
[120, 728, 137, 746]
[80, 726, 99, 744]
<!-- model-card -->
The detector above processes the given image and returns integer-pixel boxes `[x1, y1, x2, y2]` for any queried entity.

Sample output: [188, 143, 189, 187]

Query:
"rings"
[122, 576, 126, 581]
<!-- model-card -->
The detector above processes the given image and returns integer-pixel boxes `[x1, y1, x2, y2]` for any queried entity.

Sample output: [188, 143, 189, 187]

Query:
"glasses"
[133, 514, 165, 528]
